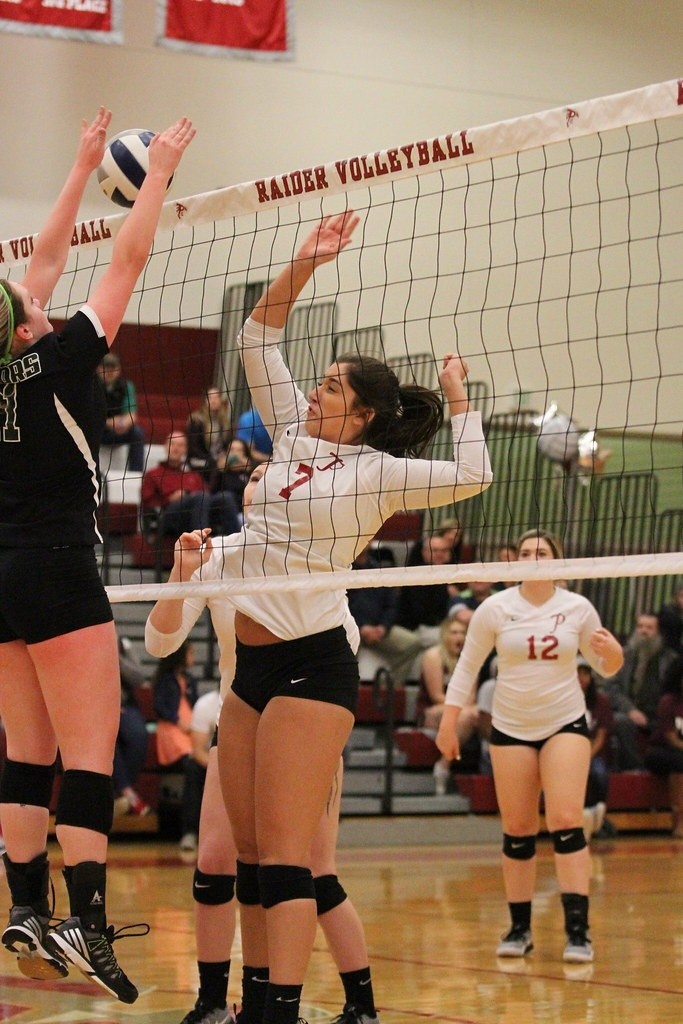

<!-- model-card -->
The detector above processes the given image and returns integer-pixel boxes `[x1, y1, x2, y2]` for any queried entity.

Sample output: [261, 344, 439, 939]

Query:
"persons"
[0, 109, 195, 1004]
[575, 586, 683, 841]
[206, 206, 493, 1023]
[94, 352, 145, 471]
[343, 516, 526, 798]
[141, 389, 274, 550]
[108, 643, 227, 852]
[434, 529, 624, 962]
[145, 463, 378, 1024]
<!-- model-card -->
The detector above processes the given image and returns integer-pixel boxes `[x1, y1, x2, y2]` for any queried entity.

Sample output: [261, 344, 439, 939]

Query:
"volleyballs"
[95, 128, 176, 208]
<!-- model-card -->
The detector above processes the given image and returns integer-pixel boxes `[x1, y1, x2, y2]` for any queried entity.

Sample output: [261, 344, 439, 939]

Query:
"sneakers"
[563, 959, 592, 980]
[181, 833, 196, 850]
[46, 917, 150, 1004]
[179, 988, 231, 1024]
[496, 924, 532, 955]
[497, 955, 530, 974]
[331, 1003, 380, 1024]
[563, 924, 593, 962]
[2, 877, 69, 980]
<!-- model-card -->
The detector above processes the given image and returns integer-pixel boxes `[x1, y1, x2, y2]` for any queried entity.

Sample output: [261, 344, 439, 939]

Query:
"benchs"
[43, 317, 683, 832]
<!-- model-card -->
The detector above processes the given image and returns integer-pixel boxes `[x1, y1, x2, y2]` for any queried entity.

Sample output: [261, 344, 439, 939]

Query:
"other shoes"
[131, 799, 151, 817]
[592, 820, 616, 838]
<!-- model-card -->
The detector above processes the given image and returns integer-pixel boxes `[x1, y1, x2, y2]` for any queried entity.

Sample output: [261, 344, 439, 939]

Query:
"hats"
[101, 353, 119, 367]
[576, 655, 591, 670]
